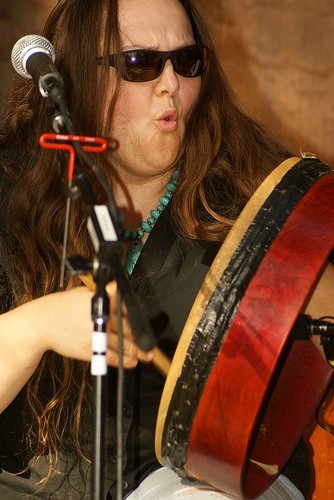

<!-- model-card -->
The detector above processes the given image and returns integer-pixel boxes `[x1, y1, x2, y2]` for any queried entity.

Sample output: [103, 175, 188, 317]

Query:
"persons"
[0, 0, 308, 500]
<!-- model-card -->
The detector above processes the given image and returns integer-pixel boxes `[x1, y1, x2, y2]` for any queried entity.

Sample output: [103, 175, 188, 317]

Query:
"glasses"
[99, 44, 211, 84]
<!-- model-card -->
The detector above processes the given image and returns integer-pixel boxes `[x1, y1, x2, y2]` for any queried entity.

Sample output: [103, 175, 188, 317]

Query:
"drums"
[156, 153, 334, 500]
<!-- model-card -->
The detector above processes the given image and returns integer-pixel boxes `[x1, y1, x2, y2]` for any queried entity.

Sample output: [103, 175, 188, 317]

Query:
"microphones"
[11, 34, 65, 104]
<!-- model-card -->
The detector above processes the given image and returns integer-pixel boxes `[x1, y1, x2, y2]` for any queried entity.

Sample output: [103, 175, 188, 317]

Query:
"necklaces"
[115, 156, 186, 278]
